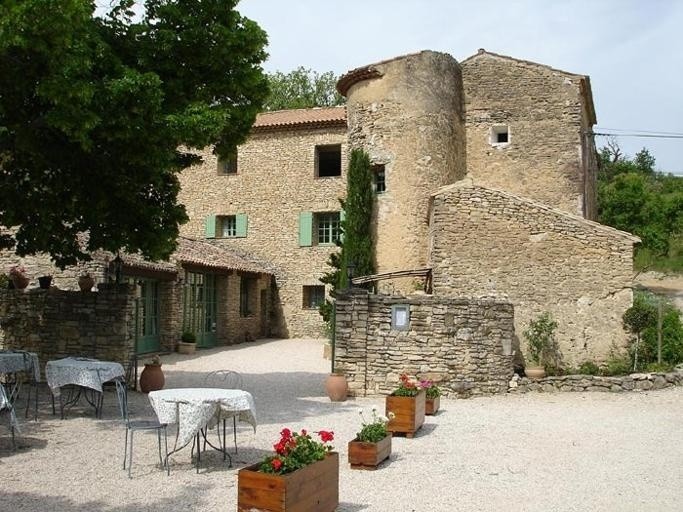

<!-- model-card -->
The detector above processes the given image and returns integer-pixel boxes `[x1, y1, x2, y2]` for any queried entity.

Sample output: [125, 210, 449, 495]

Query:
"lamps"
[106, 251, 125, 286]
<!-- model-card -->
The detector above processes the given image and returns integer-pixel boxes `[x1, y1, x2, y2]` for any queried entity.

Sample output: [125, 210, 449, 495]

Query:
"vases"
[38, 277, 52, 289]
[386, 393, 425, 438]
[238, 452, 339, 512]
[13, 278, 30, 289]
[325, 374, 347, 402]
[78, 276, 95, 293]
[139, 364, 165, 394]
[425, 396, 440, 416]
[348, 432, 392, 470]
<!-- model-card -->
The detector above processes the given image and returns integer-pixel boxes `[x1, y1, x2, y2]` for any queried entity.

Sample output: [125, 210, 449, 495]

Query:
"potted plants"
[411, 281, 426, 295]
[523, 311, 560, 378]
[178, 331, 198, 354]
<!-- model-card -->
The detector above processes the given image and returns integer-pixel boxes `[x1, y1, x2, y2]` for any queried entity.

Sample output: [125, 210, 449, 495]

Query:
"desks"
[0, 352, 39, 446]
[148, 387, 257, 475]
[44, 356, 126, 420]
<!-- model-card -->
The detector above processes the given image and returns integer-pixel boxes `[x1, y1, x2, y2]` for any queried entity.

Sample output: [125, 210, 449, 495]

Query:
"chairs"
[96, 357, 135, 420]
[22, 352, 65, 422]
[190, 369, 243, 461]
[115, 376, 170, 479]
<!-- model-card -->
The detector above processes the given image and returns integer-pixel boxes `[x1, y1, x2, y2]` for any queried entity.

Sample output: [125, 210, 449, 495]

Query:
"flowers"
[392, 373, 419, 397]
[256, 427, 335, 476]
[8, 265, 29, 280]
[356, 408, 396, 442]
[416, 374, 443, 398]
